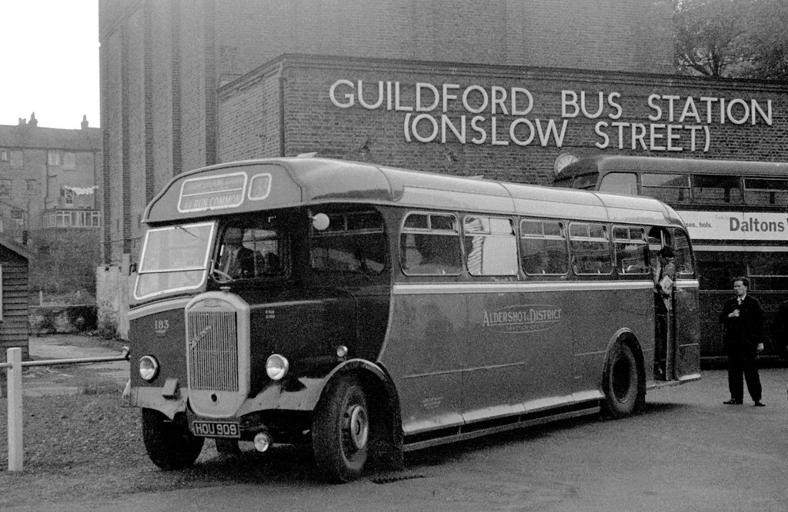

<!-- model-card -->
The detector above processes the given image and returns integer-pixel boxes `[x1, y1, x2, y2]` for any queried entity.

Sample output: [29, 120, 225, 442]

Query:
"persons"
[718, 276, 766, 406]
[649, 247, 676, 380]
[216, 223, 266, 281]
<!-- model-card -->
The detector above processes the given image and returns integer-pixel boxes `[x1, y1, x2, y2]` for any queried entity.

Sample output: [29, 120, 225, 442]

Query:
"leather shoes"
[723, 398, 742, 405]
[755, 401, 766, 406]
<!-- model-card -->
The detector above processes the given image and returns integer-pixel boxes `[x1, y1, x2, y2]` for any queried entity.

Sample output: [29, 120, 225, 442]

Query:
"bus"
[113, 157, 706, 485]
[550, 150, 788, 365]
